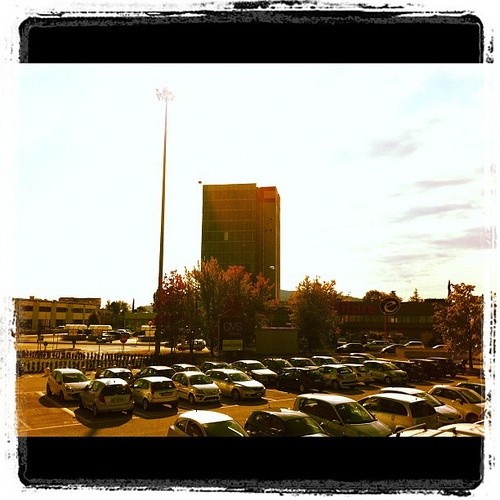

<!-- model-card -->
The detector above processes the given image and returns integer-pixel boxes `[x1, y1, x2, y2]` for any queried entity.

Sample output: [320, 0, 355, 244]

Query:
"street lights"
[155, 86, 175, 355]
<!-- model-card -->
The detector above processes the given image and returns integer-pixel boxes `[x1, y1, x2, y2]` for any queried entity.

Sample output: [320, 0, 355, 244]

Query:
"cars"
[166, 382, 485, 437]
[310, 364, 358, 390]
[362, 360, 407, 385]
[96, 361, 231, 384]
[80, 378, 135, 417]
[47, 368, 91, 403]
[205, 369, 266, 401]
[336, 338, 448, 355]
[275, 367, 324, 393]
[382, 357, 466, 382]
[229, 360, 278, 385]
[108, 329, 136, 339]
[344, 364, 373, 386]
[130, 376, 178, 410]
[171, 371, 221, 404]
[263, 352, 386, 374]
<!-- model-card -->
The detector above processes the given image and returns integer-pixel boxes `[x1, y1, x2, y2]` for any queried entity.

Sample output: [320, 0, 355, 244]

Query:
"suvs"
[177, 340, 206, 352]
